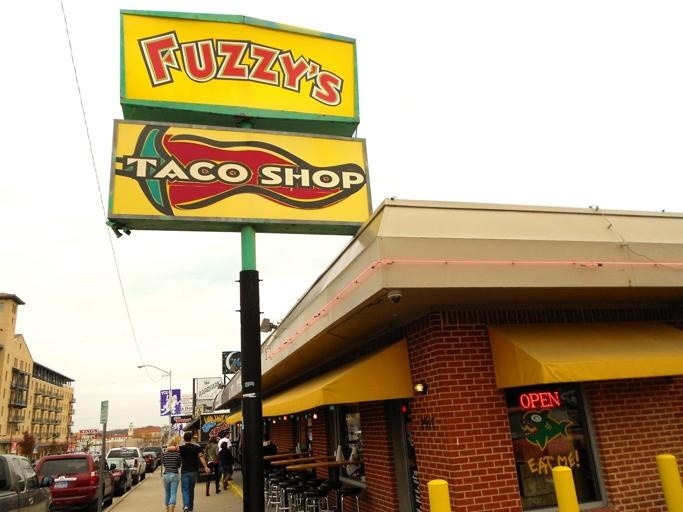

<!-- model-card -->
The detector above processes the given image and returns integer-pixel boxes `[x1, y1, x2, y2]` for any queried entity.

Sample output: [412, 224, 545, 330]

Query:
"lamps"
[258, 317, 277, 335]
[413, 381, 427, 397]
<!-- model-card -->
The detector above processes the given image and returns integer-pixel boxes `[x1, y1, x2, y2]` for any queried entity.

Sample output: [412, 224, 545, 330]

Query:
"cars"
[141, 451, 160, 472]
[0, 453, 53, 512]
[105, 457, 130, 496]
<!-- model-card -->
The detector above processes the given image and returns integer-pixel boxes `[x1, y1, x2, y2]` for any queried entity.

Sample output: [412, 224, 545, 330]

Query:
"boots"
[164, 505, 168, 512]
[169, 503, 176, 512]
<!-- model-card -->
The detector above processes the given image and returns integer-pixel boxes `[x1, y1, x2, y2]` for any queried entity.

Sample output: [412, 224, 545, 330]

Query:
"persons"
[262, 436, 281, 458]
[161, 429, 235, 512]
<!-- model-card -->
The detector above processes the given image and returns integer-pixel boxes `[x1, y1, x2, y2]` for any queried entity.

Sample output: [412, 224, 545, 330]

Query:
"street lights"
[136, 363, 172, 440]
[8, 428, 19, 453]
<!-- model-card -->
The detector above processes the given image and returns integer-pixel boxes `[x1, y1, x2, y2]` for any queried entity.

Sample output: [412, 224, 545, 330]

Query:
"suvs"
[33, 454, 116, 512]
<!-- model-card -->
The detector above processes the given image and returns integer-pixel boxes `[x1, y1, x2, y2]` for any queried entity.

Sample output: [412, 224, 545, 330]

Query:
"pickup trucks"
[105, 447, 146, 485]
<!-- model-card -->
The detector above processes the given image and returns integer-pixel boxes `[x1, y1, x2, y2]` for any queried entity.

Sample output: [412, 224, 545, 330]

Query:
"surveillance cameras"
[388, 289, 402, 303]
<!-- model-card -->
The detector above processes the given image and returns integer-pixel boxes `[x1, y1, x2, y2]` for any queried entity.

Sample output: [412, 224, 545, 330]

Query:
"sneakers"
[183, 506, 189, 512]
[206, 493, 210, 496]
[216, 489, 222, 493]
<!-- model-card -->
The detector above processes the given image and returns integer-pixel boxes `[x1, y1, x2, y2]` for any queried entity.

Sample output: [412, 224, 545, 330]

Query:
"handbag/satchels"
[212, 459, 220, 464]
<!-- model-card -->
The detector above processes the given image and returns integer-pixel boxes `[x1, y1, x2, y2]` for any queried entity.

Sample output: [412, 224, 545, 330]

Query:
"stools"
[261, 452, 366, 511]
